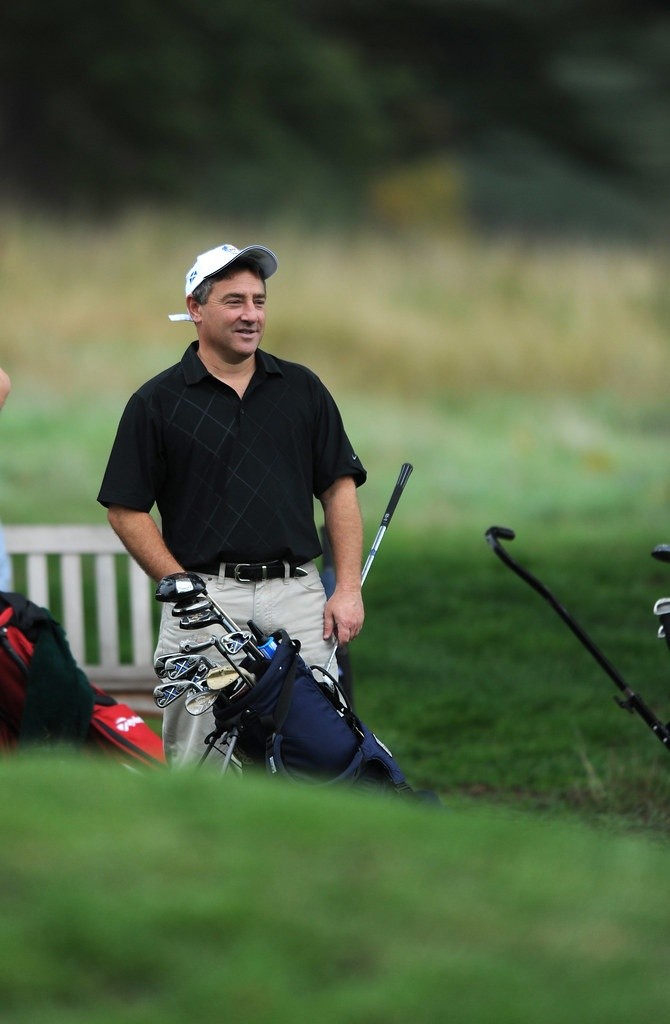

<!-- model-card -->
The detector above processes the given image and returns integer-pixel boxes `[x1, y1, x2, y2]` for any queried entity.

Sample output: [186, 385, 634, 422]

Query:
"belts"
[180, 553, 316, 583]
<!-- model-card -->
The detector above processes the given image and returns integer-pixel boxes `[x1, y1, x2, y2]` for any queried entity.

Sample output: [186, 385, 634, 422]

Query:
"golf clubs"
[649, 541, 670, 652]
[148, 571, 264, 717]
[318, 459, 414, 679]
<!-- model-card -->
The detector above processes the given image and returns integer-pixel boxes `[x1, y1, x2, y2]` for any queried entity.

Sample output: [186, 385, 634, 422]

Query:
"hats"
[185, 244, 278, 299]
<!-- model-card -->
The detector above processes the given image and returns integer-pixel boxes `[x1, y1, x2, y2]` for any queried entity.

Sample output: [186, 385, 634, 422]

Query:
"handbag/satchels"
[1, 606, 166, 782]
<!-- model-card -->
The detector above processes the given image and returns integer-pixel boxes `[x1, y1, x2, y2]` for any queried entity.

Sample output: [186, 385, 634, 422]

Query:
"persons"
[97, 244, 364, 776]
[0, 366, 10, 409]
